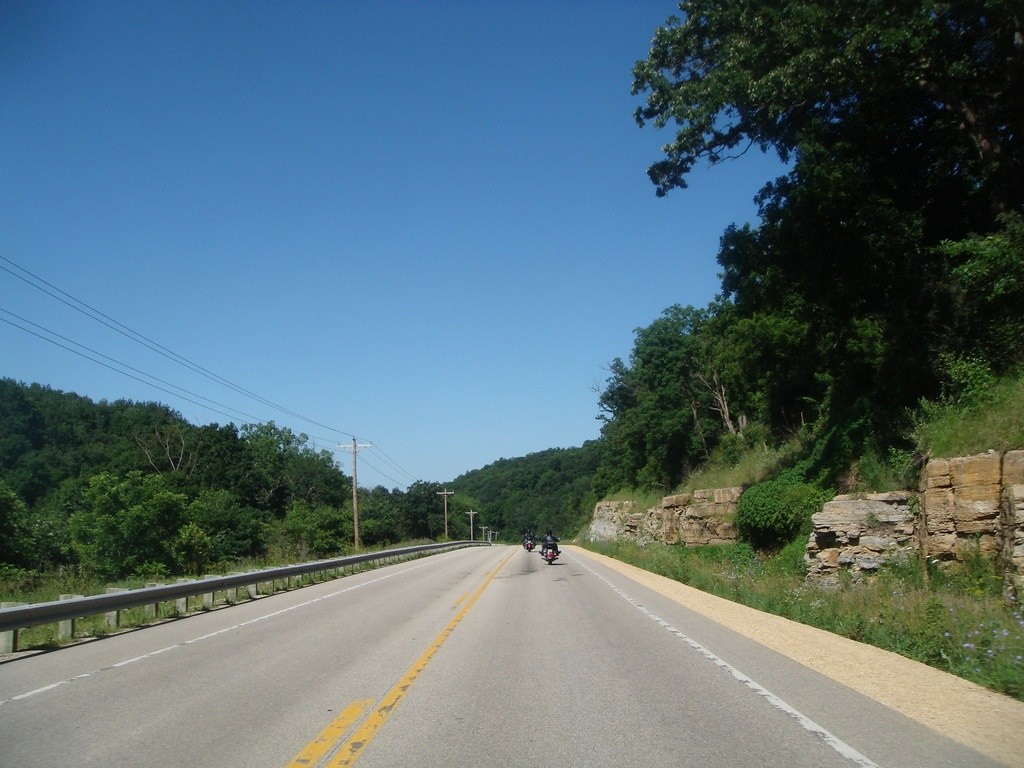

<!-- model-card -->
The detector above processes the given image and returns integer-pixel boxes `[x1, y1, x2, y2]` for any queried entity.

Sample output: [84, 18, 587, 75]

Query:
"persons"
[522, 530, 537, 549]
[539, 531, 561, 558]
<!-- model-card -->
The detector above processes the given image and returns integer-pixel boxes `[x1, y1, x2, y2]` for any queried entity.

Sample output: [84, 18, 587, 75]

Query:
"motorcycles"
[538, 537, 561, 564]
[522, 535, 537, 552]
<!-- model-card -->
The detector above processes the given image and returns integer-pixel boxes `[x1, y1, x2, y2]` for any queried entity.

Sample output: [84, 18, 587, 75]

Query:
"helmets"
[527, 529, 530, 533]
[547, 530, 552, 534]
